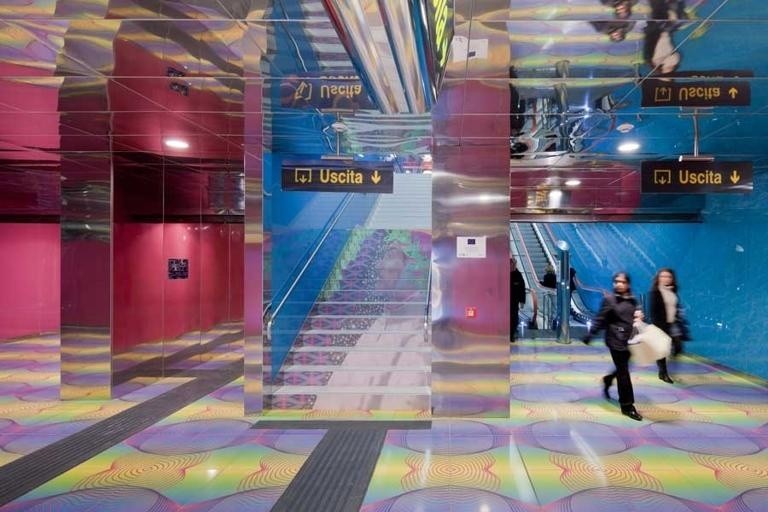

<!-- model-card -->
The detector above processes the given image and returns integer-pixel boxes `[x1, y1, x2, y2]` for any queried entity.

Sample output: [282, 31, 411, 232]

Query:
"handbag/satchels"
[627, 318, 673, 369]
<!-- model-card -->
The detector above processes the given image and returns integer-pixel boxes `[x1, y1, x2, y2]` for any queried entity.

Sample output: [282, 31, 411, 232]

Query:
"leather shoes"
[658, 371, 675, 384]
[601, 375, 613, 400]
[619, 406, 643, 421]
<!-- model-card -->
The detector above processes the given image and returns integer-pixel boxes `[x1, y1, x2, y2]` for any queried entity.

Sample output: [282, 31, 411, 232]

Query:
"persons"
[510, 84, 526, 139]
[594, 22, 637, 44]
[510, 258, 527, 342]
[539, 264, 556, 288]
[581, 270, 644, 420]
[648, 268, 682, 383]
[570, 266, 577, 298]
